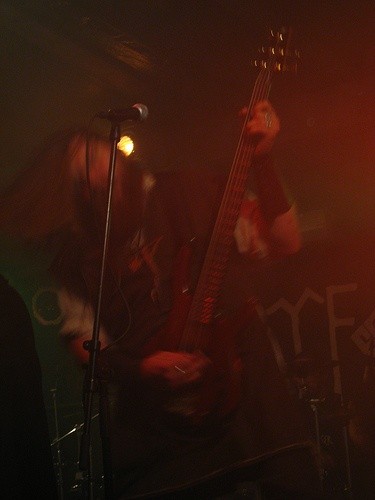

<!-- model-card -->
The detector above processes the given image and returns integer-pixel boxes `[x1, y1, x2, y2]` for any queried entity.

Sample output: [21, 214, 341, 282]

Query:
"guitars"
[138, 23, 303, 423]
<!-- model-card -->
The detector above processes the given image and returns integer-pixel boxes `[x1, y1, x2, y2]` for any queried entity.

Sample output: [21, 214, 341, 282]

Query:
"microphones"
[98, 103, 149, 121]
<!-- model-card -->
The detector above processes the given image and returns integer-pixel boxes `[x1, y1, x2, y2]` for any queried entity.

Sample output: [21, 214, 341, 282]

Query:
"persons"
[47, 97, 302, 500]
